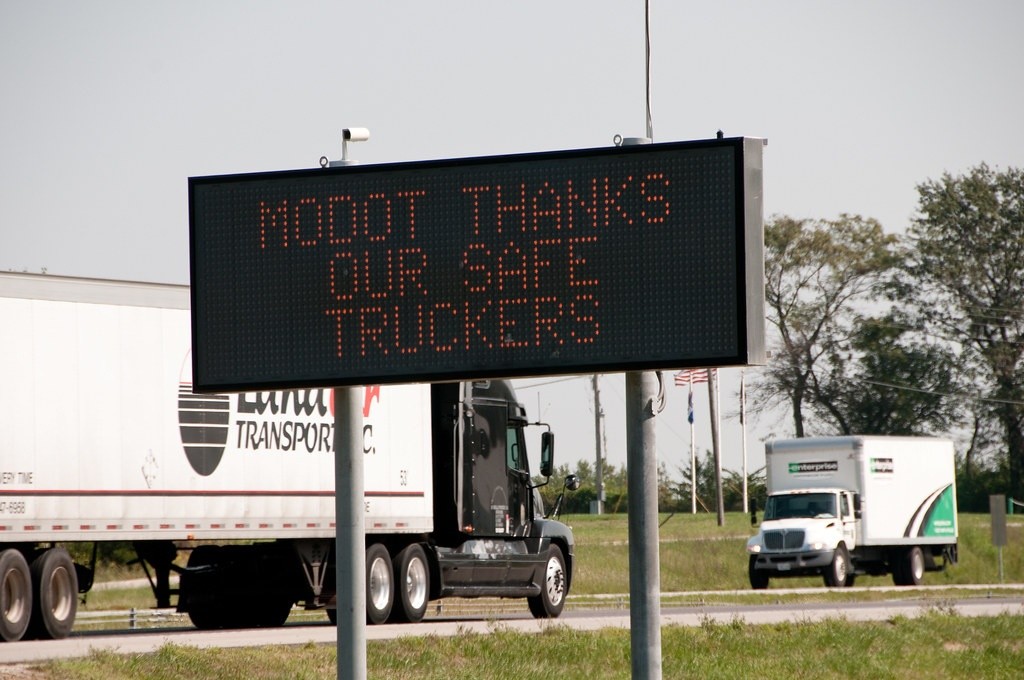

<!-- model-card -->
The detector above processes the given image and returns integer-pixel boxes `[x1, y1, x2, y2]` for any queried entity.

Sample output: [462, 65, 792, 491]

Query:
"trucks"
[746, 435, 959, 589]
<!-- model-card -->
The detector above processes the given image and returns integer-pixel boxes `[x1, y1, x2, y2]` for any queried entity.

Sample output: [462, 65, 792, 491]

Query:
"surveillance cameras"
[342, 127, 370, 141]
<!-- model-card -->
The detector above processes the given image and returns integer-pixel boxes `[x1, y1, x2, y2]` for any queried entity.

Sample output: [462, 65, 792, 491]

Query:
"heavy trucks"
[0, 271, 581, 642]
